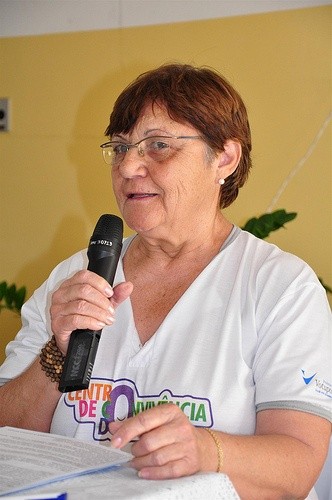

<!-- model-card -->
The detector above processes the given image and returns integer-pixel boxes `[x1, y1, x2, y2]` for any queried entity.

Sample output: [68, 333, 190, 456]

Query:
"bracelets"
[204, 427, 224, 472]
[39, 334, 66, 383]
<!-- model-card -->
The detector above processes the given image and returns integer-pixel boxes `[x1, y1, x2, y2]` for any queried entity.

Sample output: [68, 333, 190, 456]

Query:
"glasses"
[100, 135, 210, 166]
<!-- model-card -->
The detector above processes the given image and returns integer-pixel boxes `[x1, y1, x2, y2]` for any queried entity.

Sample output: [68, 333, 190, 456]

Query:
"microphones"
[58, 214, 124, 393]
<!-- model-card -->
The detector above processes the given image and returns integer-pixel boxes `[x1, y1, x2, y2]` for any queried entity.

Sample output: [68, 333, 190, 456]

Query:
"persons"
[0, 59, 331, 500]
